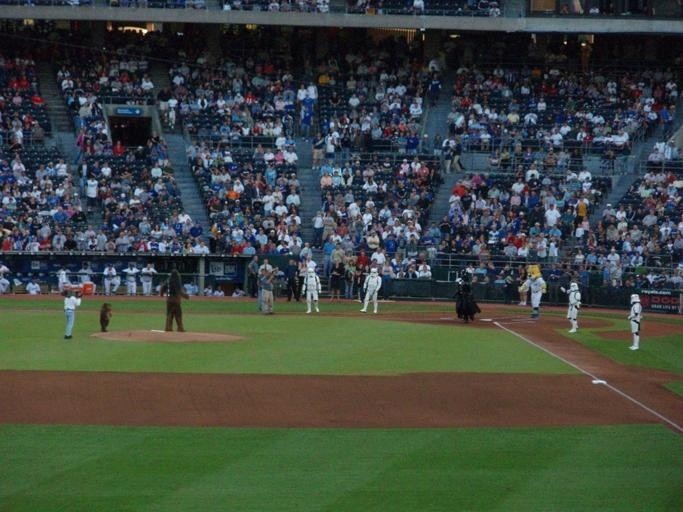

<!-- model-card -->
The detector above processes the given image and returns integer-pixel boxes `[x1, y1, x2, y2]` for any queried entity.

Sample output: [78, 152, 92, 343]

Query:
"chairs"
[0, 1, 683, 313]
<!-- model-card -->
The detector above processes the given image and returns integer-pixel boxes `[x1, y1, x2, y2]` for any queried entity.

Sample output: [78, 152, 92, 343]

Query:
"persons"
[261, 269, 274, 314]
[452, 284, 464, 320]
[283, 259, 300, 304]
[301, 268, 322, 314]
[516, 267, 529, 305]
[560, 281, 581, 332]
[328, 257, 345, 303]
[62, 289, 80, 339]
[98, 303, 112, 332]
[503, 264, 514, 305]
[627, 294, 642, 351]
[359, 268, 382, 313]
[454, 277, 480, 324]
[1, 1, 682, 296]
[255, 268, 264, 312]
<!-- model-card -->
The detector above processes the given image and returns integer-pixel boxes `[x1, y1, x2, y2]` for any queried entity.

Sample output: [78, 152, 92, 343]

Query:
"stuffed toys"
[159, 269, 189, 332]
[517, 265, 547, 319]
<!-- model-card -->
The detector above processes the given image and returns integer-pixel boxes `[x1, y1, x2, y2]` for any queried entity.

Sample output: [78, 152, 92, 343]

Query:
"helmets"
[570, 283, 579, 291]
[371, 268, 378, 276]
[630, 294, 640, 305]
[307, 268, 314, 278]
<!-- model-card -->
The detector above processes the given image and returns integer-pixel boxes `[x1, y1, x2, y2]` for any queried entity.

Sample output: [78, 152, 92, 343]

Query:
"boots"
[463, 315, 469, 324]
[314, 301, 320, 313]
[305, 303, 312, 314]
[628, 334, 640, 351]
[359, 300, 369, 313]
[373, 302, 378, 314]
[568, 319, 579, 333]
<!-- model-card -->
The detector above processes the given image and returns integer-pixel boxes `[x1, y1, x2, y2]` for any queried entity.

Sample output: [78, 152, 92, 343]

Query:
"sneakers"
[64, 334, 72, 339]
[517, 301, 527, 306]
[249, 295, 300, 316]
